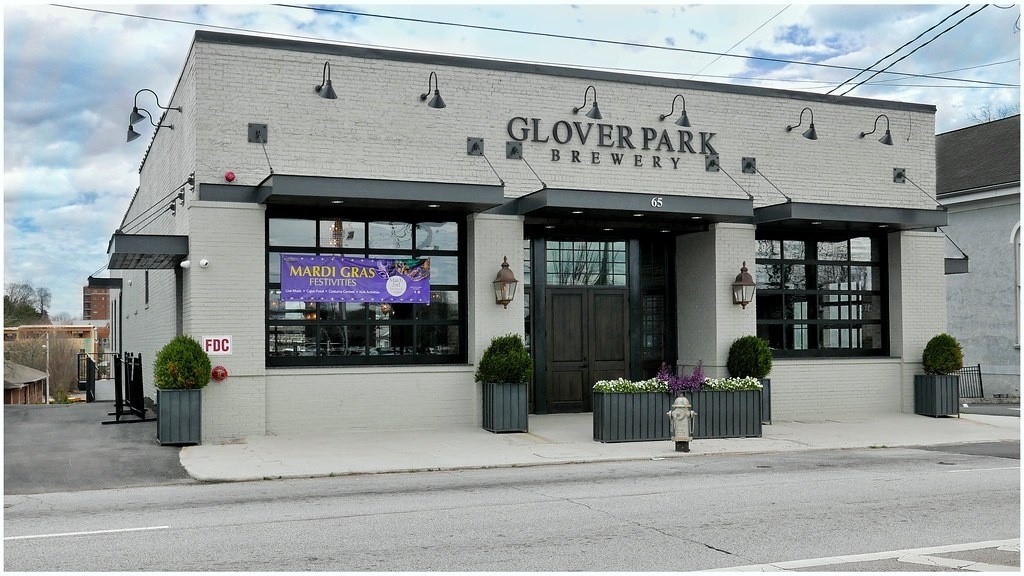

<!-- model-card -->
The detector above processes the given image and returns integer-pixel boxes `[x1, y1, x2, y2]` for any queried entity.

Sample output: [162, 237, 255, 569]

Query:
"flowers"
[590, 360, 764, 390]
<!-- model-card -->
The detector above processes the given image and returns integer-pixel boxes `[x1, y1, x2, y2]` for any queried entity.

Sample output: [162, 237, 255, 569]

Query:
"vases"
[591, 388, 763, 442]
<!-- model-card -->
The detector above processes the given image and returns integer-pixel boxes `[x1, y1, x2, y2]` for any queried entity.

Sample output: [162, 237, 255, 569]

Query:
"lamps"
[787, 108, 818, 140]
[860, 114, 894, 146]
[660, 94, 691, 127]
[732, 260, 756, 309]
[127, 108, 173, 143]
[130, 89, 182, 124]
[493, 255, 518, 309]
[421, 72, 446, 108]
[572, 86, 602, 119]
[314, 61, 337, 99]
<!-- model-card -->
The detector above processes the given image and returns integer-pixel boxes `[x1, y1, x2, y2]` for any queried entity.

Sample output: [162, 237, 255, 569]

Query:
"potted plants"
[915, 334, 964, 419]
[476, 332, 529, 433]
[728, 336, 772, 425]
[154, 334, 212, 444]
